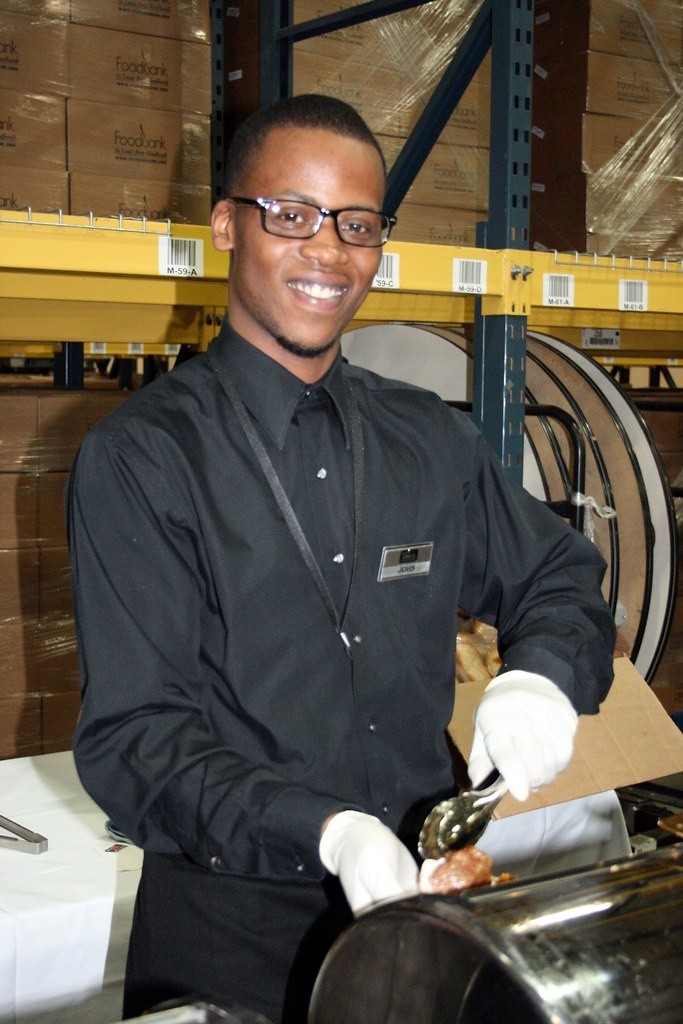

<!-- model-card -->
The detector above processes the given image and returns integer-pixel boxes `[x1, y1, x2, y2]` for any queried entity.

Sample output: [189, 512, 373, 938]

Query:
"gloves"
[320, 809, 418, 915]
[466, 670, 577, 799]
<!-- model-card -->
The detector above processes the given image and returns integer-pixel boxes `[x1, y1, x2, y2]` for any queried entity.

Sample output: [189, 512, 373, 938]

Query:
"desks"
[0, 744, 634, 1023]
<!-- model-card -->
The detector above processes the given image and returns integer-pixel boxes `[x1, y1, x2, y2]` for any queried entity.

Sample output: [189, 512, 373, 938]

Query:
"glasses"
[232, 198, 397, 248]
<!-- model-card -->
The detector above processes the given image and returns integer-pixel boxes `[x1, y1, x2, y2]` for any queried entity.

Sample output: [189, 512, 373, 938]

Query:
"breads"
[455, 617, 502, 683]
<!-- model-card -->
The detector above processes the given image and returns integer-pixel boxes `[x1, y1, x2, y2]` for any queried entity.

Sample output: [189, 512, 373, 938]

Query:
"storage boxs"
[0, 0, 683, 820]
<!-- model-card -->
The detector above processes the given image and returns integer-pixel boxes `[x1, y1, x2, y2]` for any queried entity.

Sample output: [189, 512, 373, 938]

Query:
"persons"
[67, 91, 617, 1024]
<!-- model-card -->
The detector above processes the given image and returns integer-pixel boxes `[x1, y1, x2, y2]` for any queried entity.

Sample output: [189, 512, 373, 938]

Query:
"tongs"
[416, 767, 518, 861]
[0, 815, 48, 855]
